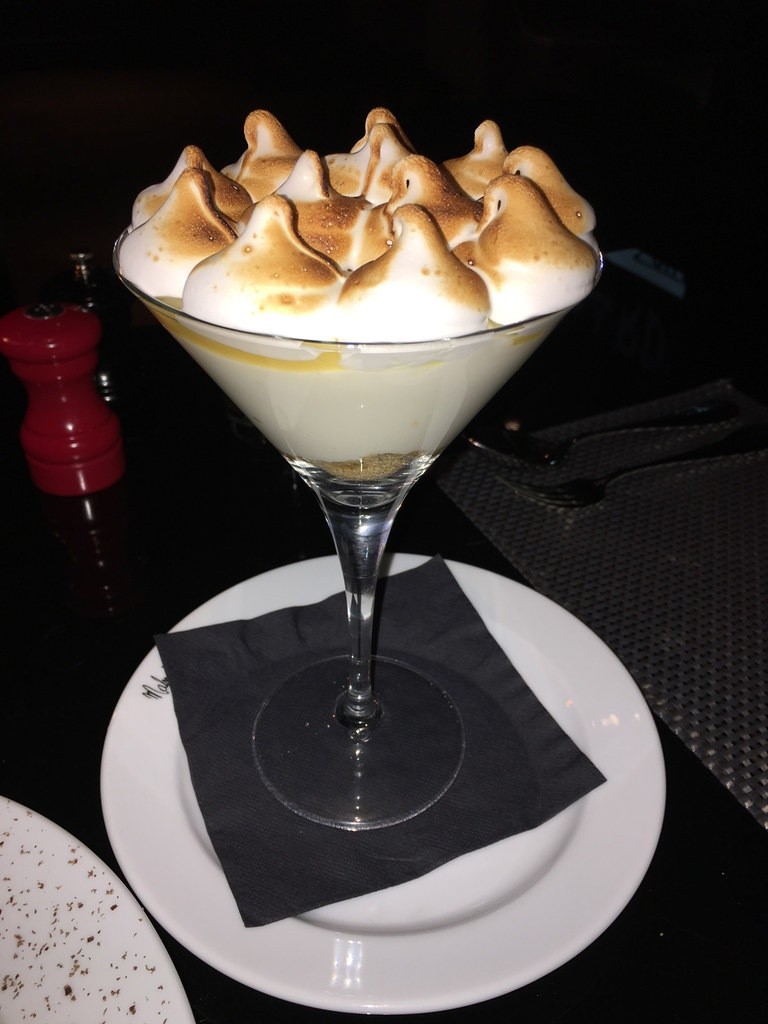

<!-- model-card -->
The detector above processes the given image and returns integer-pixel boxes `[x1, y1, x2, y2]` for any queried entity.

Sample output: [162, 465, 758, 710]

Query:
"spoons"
[469, 394, 740, 469]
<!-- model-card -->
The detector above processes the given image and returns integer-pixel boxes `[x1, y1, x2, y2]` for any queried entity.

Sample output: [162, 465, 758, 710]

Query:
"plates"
[0, 795, 195, 1024]
[100, 554, 668, 1017]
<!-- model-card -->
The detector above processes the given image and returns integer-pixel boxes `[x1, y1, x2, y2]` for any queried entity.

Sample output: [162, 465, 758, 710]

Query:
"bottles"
[68, 248, 126, 406]
[41, 495, 141, 625]
[0, 301, 126, 496]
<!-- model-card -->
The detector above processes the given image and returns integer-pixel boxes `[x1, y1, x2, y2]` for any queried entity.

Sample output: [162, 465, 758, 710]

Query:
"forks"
[494, 421, 768, 509]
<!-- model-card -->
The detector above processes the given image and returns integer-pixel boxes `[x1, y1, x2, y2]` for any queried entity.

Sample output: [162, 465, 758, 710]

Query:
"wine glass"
[111, 226, 607, 834]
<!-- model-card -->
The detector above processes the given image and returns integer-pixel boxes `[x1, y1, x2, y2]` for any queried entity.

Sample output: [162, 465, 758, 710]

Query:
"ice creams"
[116, 108, 599, 507]
